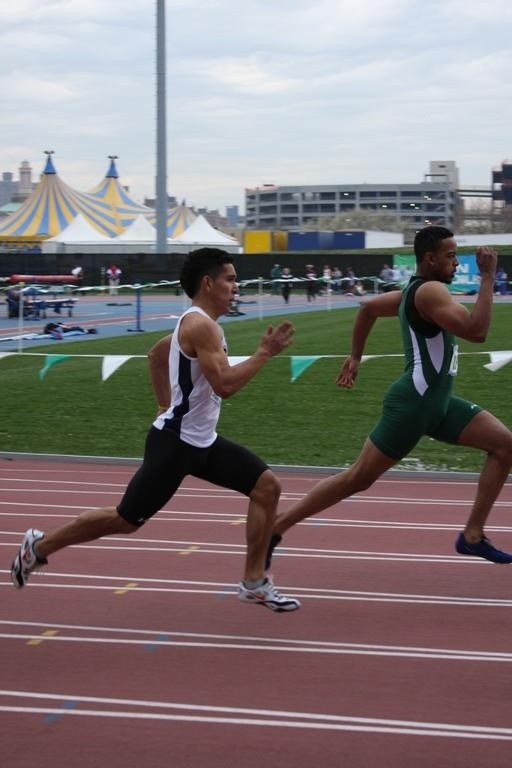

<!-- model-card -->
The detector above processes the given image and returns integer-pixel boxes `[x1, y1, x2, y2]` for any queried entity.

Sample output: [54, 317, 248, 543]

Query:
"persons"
[379, 264, 394, 284]
[265, 225, 512, 572]
[271, 263, 281, 296]
[9, 247, 304, 612]
[279, 267, 294, 304]
[305, 264, 320, 304]
[322, 264, 332, 292]
[43, 321, 98, 340]
[105, 264, 122, 295]
[332, 266, 344, 293]
[345, 266, 355, 293]
[353, 281, 367, 295]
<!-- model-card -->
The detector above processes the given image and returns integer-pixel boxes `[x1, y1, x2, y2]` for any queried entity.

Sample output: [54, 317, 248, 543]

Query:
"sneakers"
[11, 527, 48, 590]
[264, 533, 282, 571]
[455, 531, 512, 564]
[238, 576, 301, 613]
